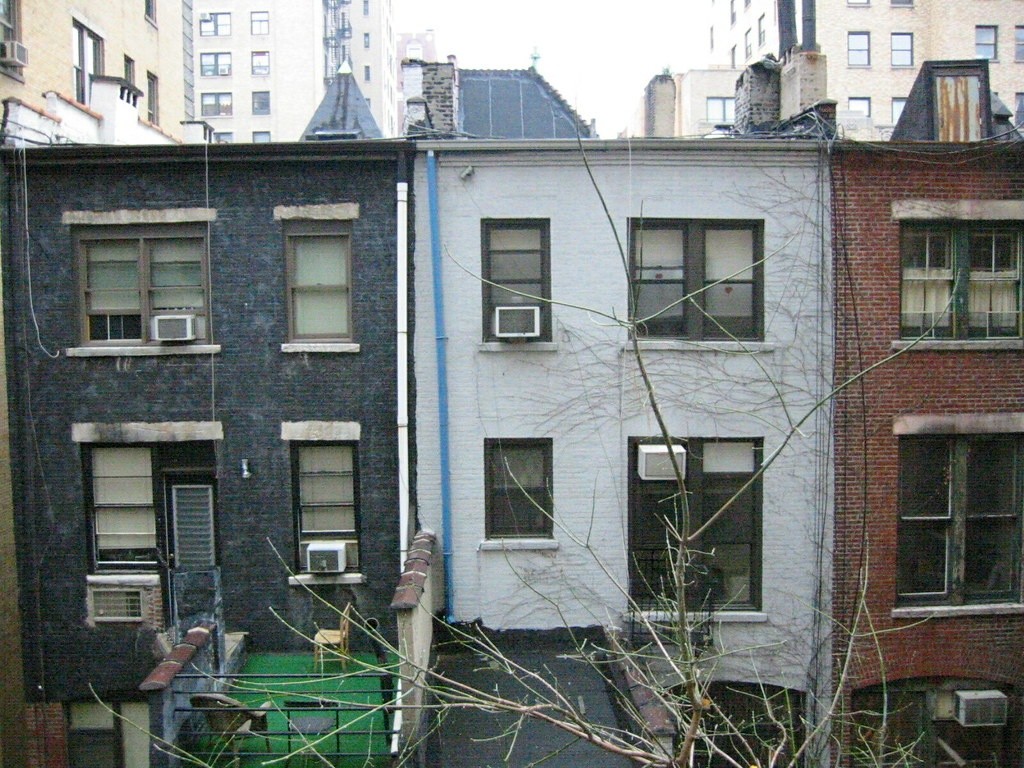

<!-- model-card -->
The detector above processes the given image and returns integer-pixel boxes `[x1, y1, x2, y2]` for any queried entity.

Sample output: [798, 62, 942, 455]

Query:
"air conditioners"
[155, 315, 197, 340]
[0, 39, 30, 70]
[90, 588, 148, 623]
[495, 306, 541, 338]
[306, 540, 346, 572]
[953, 690, 1008, 727]
[219, 66, 228, 74]
[200, 12, 211, 21]
[637, 445, 686, 481]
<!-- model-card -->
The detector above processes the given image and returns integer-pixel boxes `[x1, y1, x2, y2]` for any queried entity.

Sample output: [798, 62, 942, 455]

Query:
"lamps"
[241, 459, 253, 478]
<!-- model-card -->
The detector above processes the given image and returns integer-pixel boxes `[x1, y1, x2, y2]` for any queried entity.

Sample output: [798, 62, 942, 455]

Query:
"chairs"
[284, 698, 340, 767]
[313, 604, 351, 671]
[191, 694, 273, 768]
